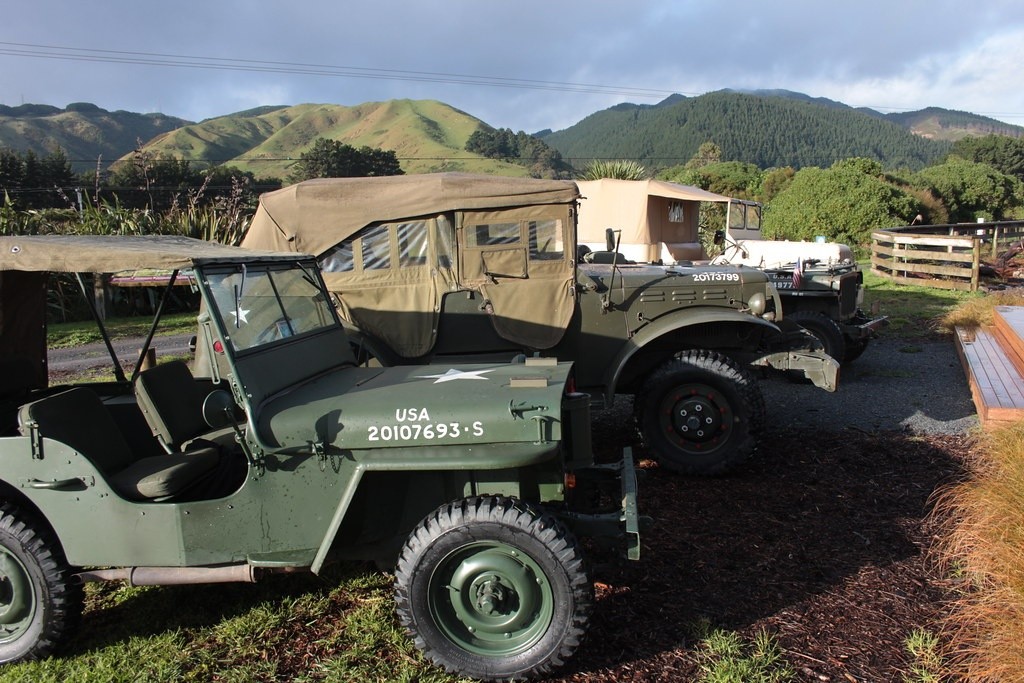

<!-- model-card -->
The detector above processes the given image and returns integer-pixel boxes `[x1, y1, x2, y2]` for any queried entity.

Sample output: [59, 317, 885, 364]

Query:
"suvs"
[440, 178, 894, 387]
[0, 230, 659, 683]
[187, 171, 844, 487]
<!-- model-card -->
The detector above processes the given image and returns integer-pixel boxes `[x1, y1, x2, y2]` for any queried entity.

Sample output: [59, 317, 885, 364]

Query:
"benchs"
[658, 241, 712, 267]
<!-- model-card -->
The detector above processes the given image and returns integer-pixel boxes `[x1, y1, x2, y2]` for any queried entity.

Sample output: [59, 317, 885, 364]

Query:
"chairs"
[133, 361, 246, 451]
[16, 386, 230, 503]
